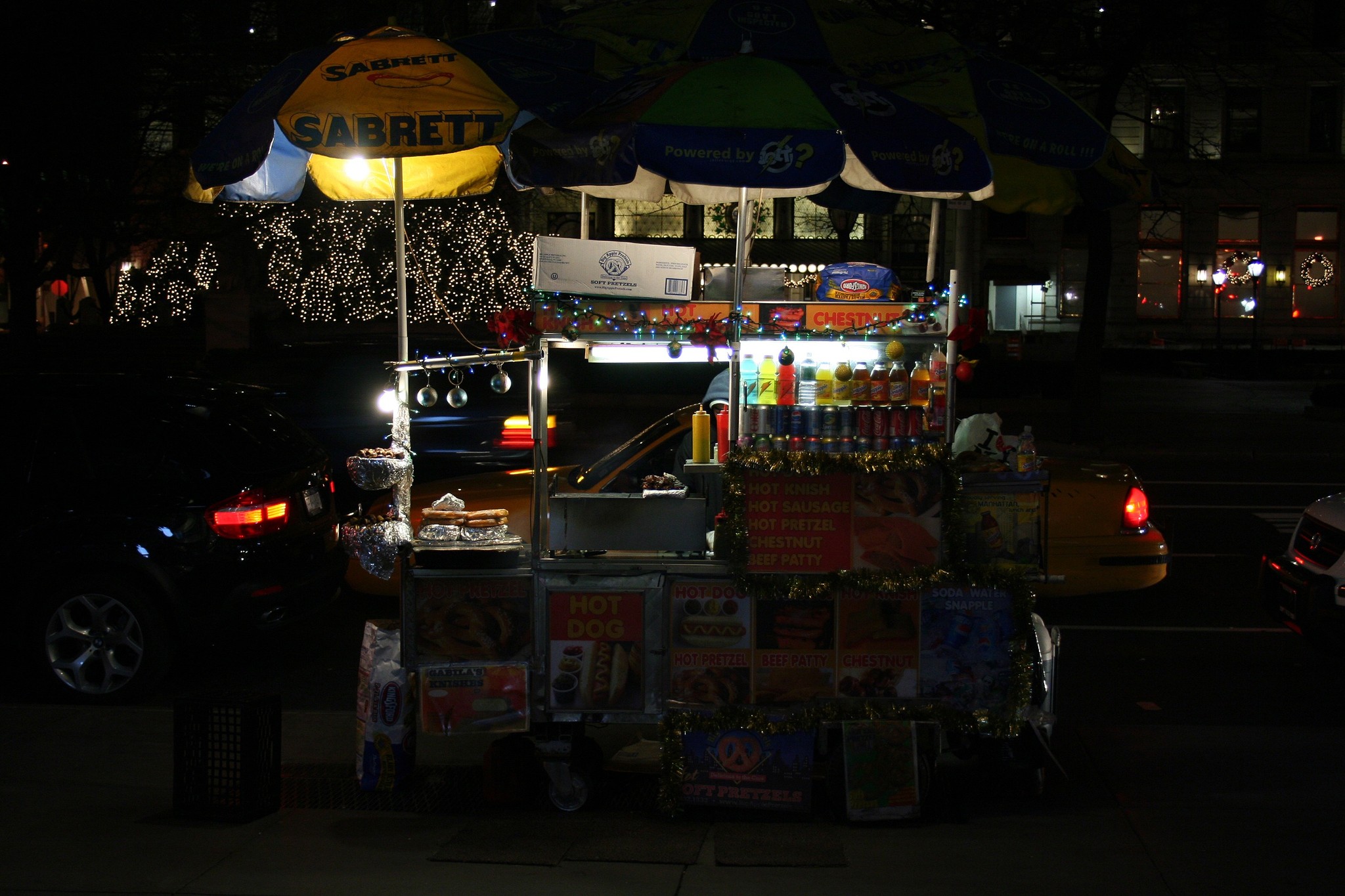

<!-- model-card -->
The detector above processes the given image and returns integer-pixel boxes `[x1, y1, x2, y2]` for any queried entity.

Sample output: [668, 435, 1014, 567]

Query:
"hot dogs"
[678, 616, 745, 648]
[578, 642, 629, 706]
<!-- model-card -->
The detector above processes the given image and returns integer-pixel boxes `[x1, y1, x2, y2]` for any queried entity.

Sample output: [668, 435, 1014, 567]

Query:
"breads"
[420, 508, 509, 527]
[417, 594, 515, 661]
[671, 666, 737, 704]
[854, 468, 932, 513]
[956, 451, 1008, 472]
[718, 736, 763, 771]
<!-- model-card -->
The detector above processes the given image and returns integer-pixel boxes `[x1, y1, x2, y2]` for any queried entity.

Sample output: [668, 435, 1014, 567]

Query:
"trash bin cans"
[172, 690, 282, 824]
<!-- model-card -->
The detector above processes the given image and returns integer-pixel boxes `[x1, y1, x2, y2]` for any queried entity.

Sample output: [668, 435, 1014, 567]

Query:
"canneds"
[740, 404, 940, 455]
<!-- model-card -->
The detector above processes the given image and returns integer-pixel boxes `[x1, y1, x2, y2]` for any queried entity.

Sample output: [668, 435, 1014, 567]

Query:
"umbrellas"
[168, 17, 519, 526]
[446, 0, 1155, 524]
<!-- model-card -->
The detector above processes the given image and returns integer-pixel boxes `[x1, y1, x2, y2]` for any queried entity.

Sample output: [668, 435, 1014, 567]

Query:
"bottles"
[1018, 425, 1038, 480]
[890, 362, 910, 406]
[853, 362, 871, 406]
[834, 362, 852, 406]
[714, 443, 717, 461]
[777, 363, 795, 406]
[714, 507, 729, 524]
[740, 354, 757, 404]
[816, 362, 834, 405]
[693, 405, 710, 463]
[717, 405, 729, 463]
[799, 352, 816, 406]
[758, 356, 777, 405]
[930, 343, 947, 405]
[910, 361, 930, 405]
[871, 362, 890, 406]
[713, 518, 730, 560]
[981, 510, 1003, 555]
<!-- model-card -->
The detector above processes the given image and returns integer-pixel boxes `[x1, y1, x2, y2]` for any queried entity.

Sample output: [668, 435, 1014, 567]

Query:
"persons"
[673, 367, 730, 529]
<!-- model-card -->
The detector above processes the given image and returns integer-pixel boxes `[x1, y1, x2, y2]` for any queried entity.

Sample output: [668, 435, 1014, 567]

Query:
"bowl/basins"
[557, 657, 583, 681]
[683, 599, 702, 616]
[562, 645, 584, 661]
[702, 598, 721, 616]
[721, 599, 739, 617]
[551, 673, 578, 703]
